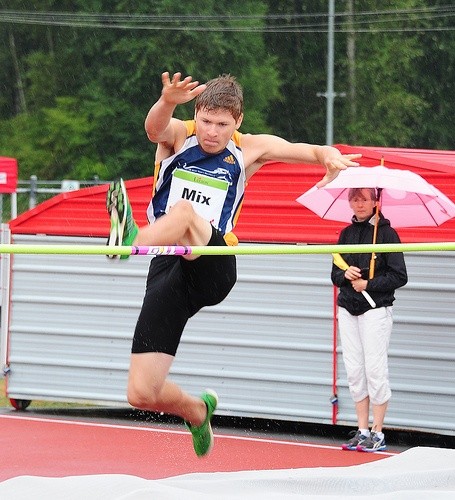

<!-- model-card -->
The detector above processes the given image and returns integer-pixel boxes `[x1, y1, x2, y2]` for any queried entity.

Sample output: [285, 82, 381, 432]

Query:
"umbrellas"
[296, 155, 454, 279]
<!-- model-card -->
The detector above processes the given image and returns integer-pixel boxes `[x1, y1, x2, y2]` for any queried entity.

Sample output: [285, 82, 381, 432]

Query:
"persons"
[331, 188, 408, 453]
[106, 73, 362, 459]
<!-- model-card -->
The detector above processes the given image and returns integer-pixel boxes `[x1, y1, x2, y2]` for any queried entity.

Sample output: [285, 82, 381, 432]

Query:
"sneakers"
[186, 389, 217, 460]
[342, 429, 369, 450]
[105, 177, 138, 261]
[355, 424, 386, 451]
